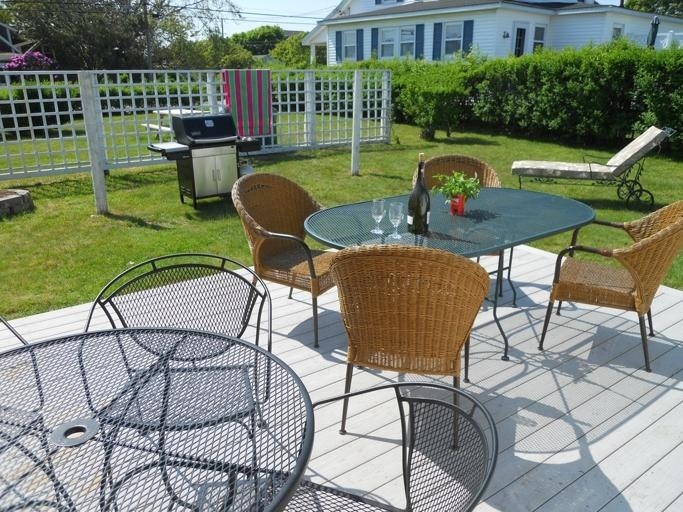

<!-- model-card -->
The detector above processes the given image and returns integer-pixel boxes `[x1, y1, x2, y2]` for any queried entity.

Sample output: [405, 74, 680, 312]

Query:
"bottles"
[405, 152, 431, 235]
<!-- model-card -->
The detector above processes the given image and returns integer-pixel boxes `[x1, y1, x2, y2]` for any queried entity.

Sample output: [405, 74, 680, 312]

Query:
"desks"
[151, 108, 210, 140]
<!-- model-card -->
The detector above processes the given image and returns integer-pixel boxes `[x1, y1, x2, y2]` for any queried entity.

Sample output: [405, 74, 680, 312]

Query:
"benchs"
[140, 123, 174, 135]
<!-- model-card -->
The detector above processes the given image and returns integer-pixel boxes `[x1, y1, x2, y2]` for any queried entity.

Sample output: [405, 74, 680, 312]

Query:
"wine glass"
[370, 197, 386, 235]
[387, 201, 405, 240]
